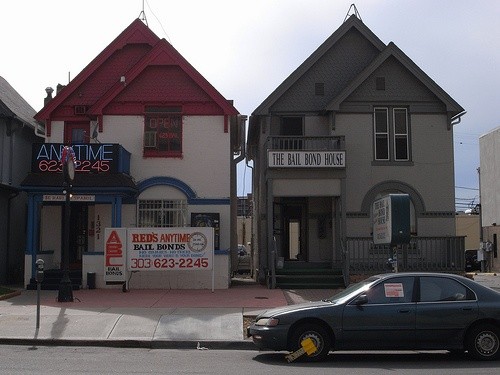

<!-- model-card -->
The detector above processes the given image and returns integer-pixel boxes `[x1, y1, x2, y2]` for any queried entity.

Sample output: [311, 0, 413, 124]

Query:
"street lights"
[58, 146, 77, 303]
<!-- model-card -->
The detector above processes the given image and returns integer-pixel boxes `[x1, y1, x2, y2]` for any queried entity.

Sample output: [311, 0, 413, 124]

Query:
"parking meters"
[35, 258, 45, 282]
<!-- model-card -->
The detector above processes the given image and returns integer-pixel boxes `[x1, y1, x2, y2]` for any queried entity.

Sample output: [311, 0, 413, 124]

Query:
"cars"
[246, 272, 500, 364]
